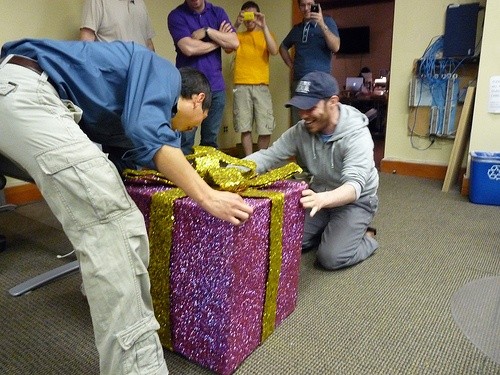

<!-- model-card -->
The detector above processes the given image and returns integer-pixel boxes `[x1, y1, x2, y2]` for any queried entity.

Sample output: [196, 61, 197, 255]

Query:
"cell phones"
[311, 5, 319, 12]
[242, 12, 255, 20]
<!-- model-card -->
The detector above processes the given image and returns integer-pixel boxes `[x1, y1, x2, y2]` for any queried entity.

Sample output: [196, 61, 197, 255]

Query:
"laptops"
[346, 77, 364, 91]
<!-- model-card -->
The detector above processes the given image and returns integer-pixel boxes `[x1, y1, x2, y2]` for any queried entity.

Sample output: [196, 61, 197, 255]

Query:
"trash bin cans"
[470, 152, 500, 206]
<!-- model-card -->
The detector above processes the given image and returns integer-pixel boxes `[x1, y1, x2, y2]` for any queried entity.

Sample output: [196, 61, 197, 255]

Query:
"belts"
[0, 56, 43, 74]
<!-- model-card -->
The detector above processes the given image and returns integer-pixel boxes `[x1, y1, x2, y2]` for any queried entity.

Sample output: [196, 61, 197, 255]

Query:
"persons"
[225, 2, 278, 157]
[78, 0, 155, 53]
[226, 71, 381, 269]
[0, 39, 254, 375]
[167, 0, 239, 154]
[280, 1, 339, 122]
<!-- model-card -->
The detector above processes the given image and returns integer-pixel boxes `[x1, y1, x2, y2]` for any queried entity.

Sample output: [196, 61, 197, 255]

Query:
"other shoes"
[367, 227, 376, 235]
[293, 172, 309, 180]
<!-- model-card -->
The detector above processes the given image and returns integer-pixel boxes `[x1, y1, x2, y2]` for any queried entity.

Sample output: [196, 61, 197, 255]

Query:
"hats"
[285, 70, 339, 109]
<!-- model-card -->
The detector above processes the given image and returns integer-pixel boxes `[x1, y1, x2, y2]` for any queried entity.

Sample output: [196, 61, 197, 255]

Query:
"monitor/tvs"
[338, 26, 370, 54]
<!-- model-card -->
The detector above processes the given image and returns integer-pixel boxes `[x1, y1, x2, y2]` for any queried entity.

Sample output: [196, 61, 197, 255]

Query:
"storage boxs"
[122, 146, 308, 375]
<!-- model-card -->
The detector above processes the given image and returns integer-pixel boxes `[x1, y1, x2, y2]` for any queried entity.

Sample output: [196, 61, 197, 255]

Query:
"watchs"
[321, 25, 328, 33]
[203, 24, 209, 37]
